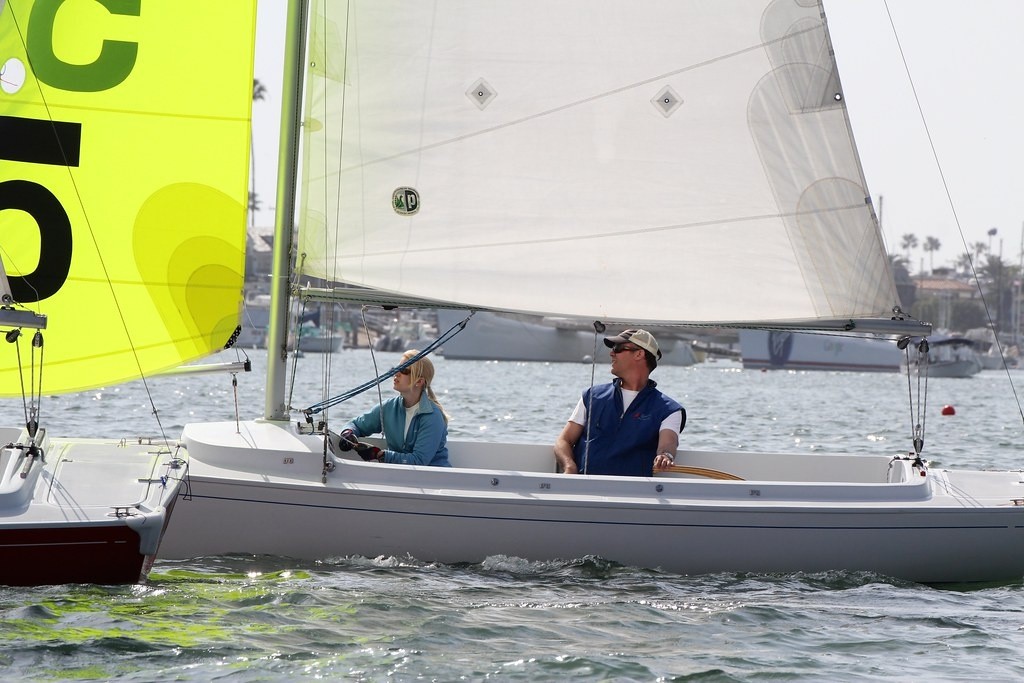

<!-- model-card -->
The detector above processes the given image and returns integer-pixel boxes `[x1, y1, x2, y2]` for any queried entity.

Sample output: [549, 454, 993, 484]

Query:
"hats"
[603, 329, 662, 361]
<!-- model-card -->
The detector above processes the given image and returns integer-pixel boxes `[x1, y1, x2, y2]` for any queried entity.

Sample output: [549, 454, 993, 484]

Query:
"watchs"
[657, 453, 674, 462]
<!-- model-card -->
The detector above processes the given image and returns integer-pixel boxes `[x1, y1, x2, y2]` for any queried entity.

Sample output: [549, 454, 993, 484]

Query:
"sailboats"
[0, 0, 257, 592]
[151, 1, 1024, 595]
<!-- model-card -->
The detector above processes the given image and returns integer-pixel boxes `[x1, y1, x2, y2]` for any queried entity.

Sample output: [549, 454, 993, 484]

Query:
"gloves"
[353, 441, 381, 461]
[339, 430, 358, 452]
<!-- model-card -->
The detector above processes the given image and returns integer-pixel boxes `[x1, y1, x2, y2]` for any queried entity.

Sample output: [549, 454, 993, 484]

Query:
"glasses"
[400, 368, 412, 375]
[613, 343, 641, 354]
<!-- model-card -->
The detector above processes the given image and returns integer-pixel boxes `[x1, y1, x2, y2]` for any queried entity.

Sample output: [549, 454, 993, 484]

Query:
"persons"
[339, 349, 452, 467]
[553, 329, 686, 477]
